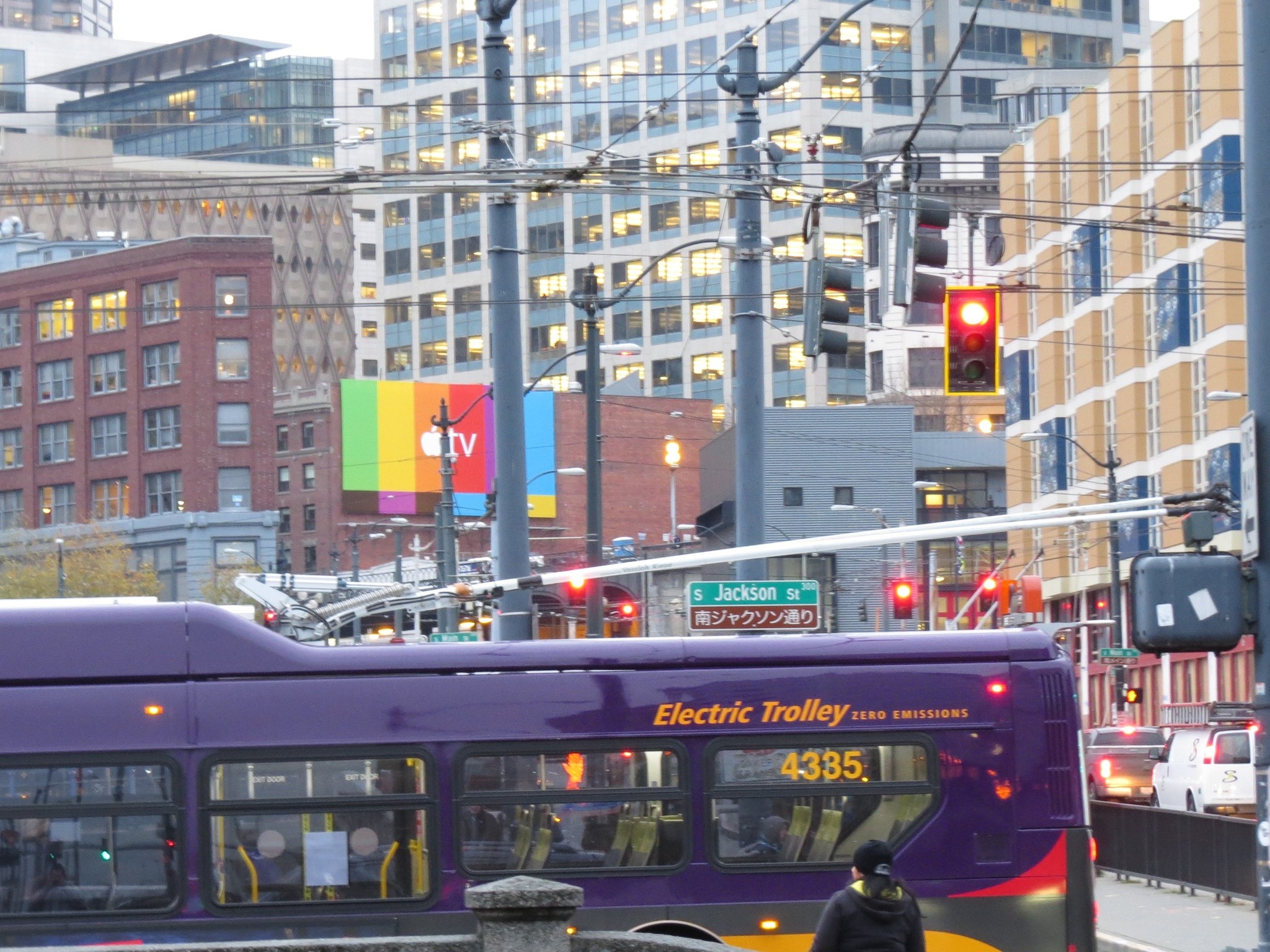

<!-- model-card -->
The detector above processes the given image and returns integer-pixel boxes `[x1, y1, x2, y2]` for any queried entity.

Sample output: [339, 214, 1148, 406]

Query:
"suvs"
[1081, 726, 1168, 804]
[1147, 724, 1266, 814]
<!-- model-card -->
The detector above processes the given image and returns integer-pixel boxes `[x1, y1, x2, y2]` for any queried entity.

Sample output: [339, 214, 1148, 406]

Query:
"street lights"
[912, 478, 966, 624]
[1018, 430, 1131, 730]
[482, 466, 587, 636]
[801, 551, 820, 585]
[832, 503, 907, 580]
[568, 230, 774, 639]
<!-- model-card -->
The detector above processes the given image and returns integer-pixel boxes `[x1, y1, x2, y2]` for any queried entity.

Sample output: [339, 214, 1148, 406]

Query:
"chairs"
[506, 804, 841, 870]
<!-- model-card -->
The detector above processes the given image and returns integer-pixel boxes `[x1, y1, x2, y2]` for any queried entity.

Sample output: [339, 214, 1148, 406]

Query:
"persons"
[808, 839, 926, 952]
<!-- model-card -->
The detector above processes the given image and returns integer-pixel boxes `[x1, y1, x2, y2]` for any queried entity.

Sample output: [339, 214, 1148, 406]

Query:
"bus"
[0, 597, 1096, 951]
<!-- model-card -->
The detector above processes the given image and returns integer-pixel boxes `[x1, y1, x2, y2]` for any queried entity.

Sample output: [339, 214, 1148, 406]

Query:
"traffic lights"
[620, 602, 635, 624]
[942, 283, 1020, 408]
[978, 573, 1002, 615]
[887, 195, 981, 328]
[801, 261, 866, 363]
[264, 610, 278, 630]
[830, 607, 840, 633]
[892, 581, 919, 622]
[1126, 688, 1144, 704]
[857, 599, 871, 622]
[570, 578, 587, 605]
